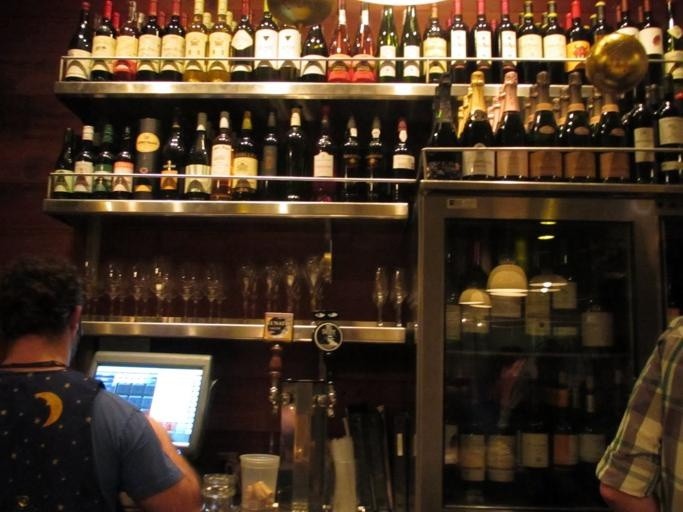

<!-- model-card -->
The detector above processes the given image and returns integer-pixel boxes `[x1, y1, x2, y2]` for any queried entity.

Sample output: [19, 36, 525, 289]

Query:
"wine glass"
[79, 255, 409, 330]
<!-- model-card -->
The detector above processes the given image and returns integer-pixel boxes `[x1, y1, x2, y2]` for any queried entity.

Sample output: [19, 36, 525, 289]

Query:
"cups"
[239, 453, 281, 510]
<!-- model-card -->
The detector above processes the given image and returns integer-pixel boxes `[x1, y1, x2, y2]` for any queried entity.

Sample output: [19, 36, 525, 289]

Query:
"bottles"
[61, 0, 683, 87]
[423, 70, 682, 184]
[443, 370, 608, 499]
[50, 105, 417, 201]
[444, 234, 617, 353]
[199, 472, 240, 510]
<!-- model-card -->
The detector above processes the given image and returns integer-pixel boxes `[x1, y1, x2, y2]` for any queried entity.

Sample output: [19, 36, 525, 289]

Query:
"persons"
[0, 267, 204, 511]
[594, 314, 681, 512]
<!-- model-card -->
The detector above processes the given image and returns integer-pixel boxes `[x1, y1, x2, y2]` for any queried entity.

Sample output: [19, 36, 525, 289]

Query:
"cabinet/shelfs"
[42, 80, 678, 344]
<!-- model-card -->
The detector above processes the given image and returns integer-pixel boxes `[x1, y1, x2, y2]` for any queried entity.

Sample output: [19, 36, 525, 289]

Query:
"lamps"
[458, 258, 568, 309]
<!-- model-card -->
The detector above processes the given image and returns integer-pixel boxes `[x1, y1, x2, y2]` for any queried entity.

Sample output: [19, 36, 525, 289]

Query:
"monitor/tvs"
[87, 351, 213, 455]
